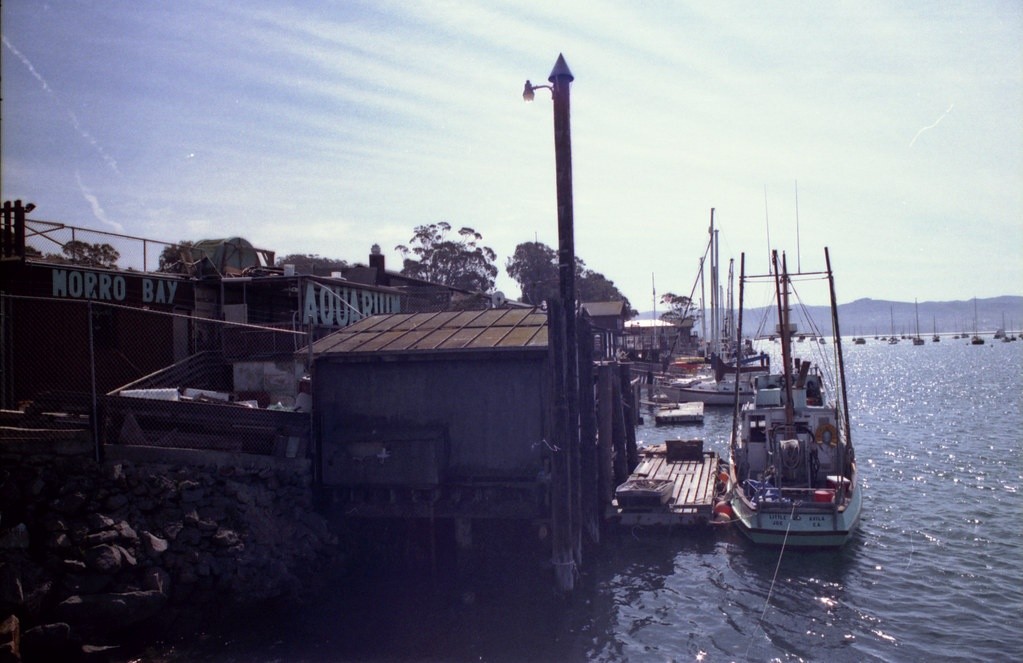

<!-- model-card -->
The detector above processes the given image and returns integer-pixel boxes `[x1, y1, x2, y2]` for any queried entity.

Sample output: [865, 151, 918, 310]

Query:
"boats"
[797, 334, 827, 344]
[724, 180, 864, 548]
[712, 258, 765, 364]
[855, 338, 865, 344]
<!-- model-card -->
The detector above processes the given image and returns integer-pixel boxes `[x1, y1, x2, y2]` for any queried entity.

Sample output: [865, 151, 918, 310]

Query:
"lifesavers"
[817, 425, 839, 447]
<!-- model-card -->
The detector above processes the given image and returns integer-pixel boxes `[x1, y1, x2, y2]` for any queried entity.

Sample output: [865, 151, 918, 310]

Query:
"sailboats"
[874, 304, 897, 344]
[971, 297, 984, 344]
[953, 316, 969, 339]
[913, 297, 923, 345]
[994, 310, 1017, 342]
[900, 318, 913, 339]
[660, 203, 768, 405]
[932, 317, 940, 341]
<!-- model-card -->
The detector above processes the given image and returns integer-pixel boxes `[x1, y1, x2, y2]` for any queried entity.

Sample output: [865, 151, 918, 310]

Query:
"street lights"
[524, 49, 581, 545]
